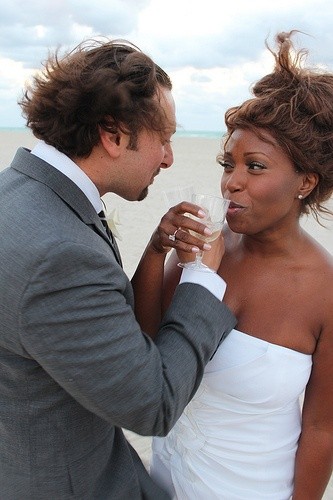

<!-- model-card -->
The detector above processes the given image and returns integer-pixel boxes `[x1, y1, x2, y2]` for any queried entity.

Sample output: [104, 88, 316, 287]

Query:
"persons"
[130, 29, 333, 500]
[0, 38, 226, 500]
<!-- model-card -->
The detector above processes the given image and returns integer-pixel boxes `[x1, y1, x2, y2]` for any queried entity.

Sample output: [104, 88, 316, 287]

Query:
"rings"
[168, 227, 182, 242]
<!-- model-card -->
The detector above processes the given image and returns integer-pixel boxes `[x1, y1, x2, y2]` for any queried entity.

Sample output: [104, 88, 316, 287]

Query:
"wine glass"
[177, 193, 232, 272]
